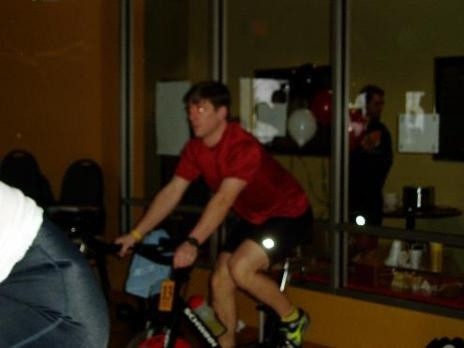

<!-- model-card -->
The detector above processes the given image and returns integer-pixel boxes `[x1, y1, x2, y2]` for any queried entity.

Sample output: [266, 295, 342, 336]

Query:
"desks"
[381, 207, 462, 232]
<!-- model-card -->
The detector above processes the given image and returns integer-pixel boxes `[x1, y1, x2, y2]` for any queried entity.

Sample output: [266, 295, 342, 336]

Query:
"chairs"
[48, 159, 110, 293]
[1, 150, 54, 214]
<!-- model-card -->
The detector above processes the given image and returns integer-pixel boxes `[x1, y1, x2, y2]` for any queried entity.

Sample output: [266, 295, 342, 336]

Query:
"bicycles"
[111, 236, 286, 347]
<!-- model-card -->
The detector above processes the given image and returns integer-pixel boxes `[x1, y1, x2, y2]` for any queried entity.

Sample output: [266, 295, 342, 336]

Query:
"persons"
[349, 85, 393, 215]
[0, 149, 110, 348]
[115, 79, 314, 348]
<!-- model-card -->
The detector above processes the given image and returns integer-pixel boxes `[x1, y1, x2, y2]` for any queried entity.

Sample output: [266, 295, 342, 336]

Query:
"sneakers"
[279, 308, 309, 347]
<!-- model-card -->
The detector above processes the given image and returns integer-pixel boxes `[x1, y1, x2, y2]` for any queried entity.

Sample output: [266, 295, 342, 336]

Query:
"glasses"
[188, 105, 207, 115]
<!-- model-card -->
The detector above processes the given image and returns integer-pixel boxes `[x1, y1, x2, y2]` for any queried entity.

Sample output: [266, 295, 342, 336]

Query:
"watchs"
[185, 236, 201, 249]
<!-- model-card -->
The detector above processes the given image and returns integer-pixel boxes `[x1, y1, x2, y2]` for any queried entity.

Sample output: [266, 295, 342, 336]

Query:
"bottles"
[188, 294, 226, 338]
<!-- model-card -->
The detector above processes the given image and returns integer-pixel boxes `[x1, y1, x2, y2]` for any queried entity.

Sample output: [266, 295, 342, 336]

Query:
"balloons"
[349, 110, 367, 150]
[310, 88, 332, 128]
[287, 108, 319, 147]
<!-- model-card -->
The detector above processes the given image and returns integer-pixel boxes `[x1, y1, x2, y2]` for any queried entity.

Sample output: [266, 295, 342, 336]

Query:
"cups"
[384, 239, 402, 267]
[409, 250, 421, 269]
[429, 242, 443, 273]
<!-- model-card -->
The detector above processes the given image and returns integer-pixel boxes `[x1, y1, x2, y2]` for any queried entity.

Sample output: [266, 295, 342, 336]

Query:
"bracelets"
[130, 229, 143, 241]
